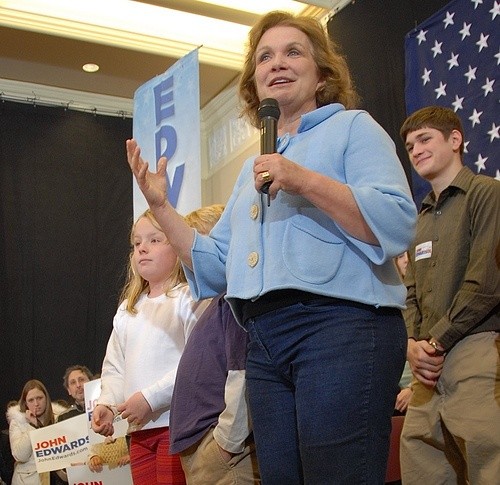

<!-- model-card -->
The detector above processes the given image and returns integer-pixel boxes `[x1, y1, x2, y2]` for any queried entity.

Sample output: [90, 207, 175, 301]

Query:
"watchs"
[425, 341, 446, 357]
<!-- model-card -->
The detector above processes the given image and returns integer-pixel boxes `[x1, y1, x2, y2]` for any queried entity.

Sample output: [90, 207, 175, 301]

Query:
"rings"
[262, 171, 271, 182]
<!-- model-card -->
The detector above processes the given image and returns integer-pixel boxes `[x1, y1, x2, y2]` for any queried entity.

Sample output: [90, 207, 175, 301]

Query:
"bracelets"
[406, 383, 413, 391]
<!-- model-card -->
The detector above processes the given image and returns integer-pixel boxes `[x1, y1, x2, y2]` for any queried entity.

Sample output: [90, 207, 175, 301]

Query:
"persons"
[0, 365, 103, 485]
[169, 203, 261, 485]
[127, 11, 417, 485]
[90, 208, 212, 485]
[393, 104, 500, 485]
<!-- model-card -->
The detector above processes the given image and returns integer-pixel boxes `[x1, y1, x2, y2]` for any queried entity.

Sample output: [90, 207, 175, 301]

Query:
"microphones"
[258, 97, 281, 207]
